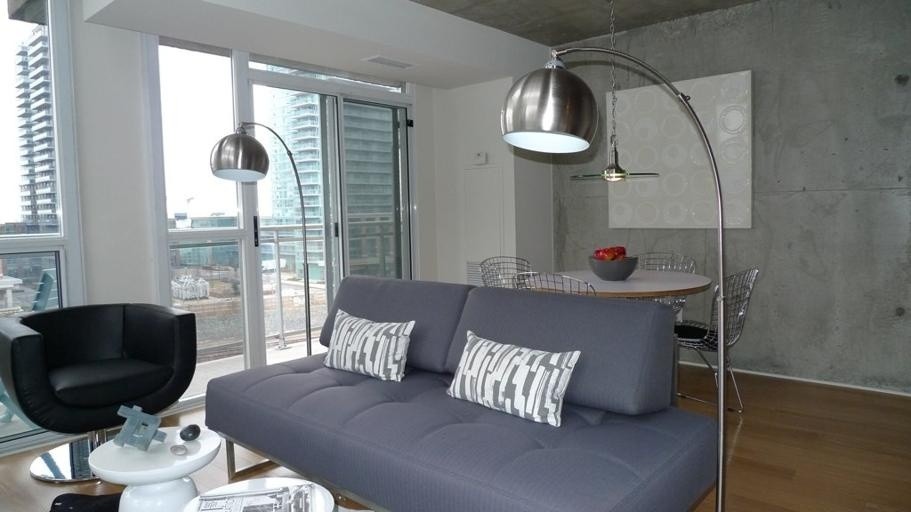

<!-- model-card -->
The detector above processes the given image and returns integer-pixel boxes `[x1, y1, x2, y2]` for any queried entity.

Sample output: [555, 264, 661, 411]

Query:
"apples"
[593, 245, 627, 261]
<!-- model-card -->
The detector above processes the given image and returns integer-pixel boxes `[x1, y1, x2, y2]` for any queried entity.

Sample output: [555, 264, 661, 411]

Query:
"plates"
[170, 445, 187, 457]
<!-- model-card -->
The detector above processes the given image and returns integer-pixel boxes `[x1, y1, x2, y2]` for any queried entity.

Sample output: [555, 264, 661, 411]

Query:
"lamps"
[210, 120, 314, 356]
[498, 42, 730, 511]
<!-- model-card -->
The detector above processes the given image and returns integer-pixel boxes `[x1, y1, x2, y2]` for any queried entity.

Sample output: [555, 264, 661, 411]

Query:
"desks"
[523, 270, 713, 299]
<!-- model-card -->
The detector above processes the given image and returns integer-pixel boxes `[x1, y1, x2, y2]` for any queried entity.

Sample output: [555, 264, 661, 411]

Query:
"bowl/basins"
[588, 259, 638, 281]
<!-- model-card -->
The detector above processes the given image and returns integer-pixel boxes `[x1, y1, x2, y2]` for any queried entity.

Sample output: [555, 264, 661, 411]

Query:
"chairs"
[2, 301, 198, 484]
[478, 254, 532, 289]
[671, 268, 760, 414]
[510, 270, 596, 296]
[628, 254, 697, 316]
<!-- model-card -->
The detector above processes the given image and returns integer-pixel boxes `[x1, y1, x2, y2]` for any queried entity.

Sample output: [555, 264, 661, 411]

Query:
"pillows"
[448, 333, 580, 428]
[323, 308, 415, 383]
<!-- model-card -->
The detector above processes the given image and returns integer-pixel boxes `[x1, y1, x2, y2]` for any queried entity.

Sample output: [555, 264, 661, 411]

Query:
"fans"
[572, 2, 661, 183]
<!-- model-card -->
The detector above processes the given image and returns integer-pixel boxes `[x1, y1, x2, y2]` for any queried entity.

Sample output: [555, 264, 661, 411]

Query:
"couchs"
[203, 274, 723, 510]
